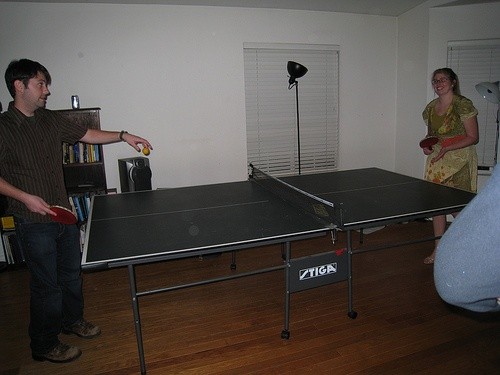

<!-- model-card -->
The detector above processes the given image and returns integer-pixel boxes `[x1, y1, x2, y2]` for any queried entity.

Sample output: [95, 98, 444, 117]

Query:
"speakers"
[119, 157, 151, 193]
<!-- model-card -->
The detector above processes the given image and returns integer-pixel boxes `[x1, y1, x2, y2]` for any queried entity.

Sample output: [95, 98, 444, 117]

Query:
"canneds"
[71, 95, 79, 110]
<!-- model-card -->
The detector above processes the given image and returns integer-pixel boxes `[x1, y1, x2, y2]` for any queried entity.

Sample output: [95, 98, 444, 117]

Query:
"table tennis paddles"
[48, 206, 78, 225]
[419, 136, 439, 150]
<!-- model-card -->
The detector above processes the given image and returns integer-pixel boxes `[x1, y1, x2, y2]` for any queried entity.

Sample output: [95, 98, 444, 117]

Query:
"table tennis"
[143, 148, 150, 155]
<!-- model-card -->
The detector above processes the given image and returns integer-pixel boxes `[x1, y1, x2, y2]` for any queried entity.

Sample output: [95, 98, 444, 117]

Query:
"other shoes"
[32, 342, 82, 363]
[61, 319, 102, 339]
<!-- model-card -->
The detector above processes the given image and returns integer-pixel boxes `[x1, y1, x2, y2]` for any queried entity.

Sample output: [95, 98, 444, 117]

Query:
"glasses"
[432, 78, 450, 85]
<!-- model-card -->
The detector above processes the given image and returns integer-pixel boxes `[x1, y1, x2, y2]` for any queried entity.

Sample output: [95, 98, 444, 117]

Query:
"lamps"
[287, 61, 309, 175]
[475, 81, 500, 166]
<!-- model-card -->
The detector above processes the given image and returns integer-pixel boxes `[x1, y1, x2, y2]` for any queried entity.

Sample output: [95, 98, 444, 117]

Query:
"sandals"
[424, 256, 434, 265]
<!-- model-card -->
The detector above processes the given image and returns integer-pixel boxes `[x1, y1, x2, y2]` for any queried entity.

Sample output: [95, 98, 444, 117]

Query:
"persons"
[421, 68, 480, 263]
[434, 161, 500, 312]
[0, 58, 153, 363]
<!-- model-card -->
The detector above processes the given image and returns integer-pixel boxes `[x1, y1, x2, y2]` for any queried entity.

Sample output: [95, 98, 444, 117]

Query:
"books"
[63, 142, 99, 164]
[68, 191, 94, 221]
[0, 232, 25, 264]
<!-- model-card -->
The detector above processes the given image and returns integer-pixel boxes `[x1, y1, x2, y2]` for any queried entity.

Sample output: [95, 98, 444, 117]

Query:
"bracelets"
[120, 130, 128, 142]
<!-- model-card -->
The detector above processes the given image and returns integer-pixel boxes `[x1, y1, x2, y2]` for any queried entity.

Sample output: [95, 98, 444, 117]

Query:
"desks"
[81, 163, 477, 375]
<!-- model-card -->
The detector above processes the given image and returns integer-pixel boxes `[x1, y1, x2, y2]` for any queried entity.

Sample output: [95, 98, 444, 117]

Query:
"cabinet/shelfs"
[0, 108, 106, 266]
